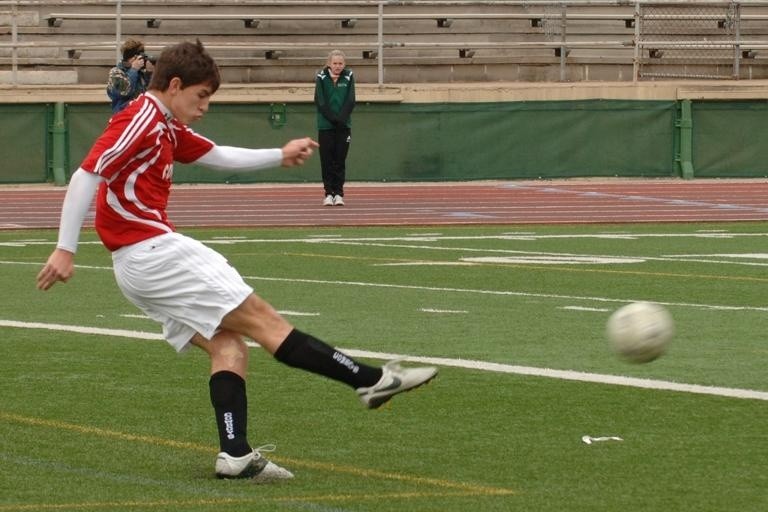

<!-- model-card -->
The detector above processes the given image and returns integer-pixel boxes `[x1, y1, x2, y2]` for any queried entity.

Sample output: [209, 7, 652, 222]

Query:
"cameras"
[139, 55, 149, 69]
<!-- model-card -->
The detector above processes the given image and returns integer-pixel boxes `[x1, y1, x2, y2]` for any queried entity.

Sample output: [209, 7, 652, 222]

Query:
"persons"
[35, 39, 440, 483]
[107, 40, 156, 116]
[315, 50, 355, 205]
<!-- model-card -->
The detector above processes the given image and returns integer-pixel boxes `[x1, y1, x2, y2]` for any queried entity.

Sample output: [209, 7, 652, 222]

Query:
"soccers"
[607, 300, 673, 365]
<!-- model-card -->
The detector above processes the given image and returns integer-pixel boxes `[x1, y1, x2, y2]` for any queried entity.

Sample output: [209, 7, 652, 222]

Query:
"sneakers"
[334, 194, 344, 206]
[213, 449, 296, 481]
[356, 362, 438, 410]
[323, 194, 334, 207]
